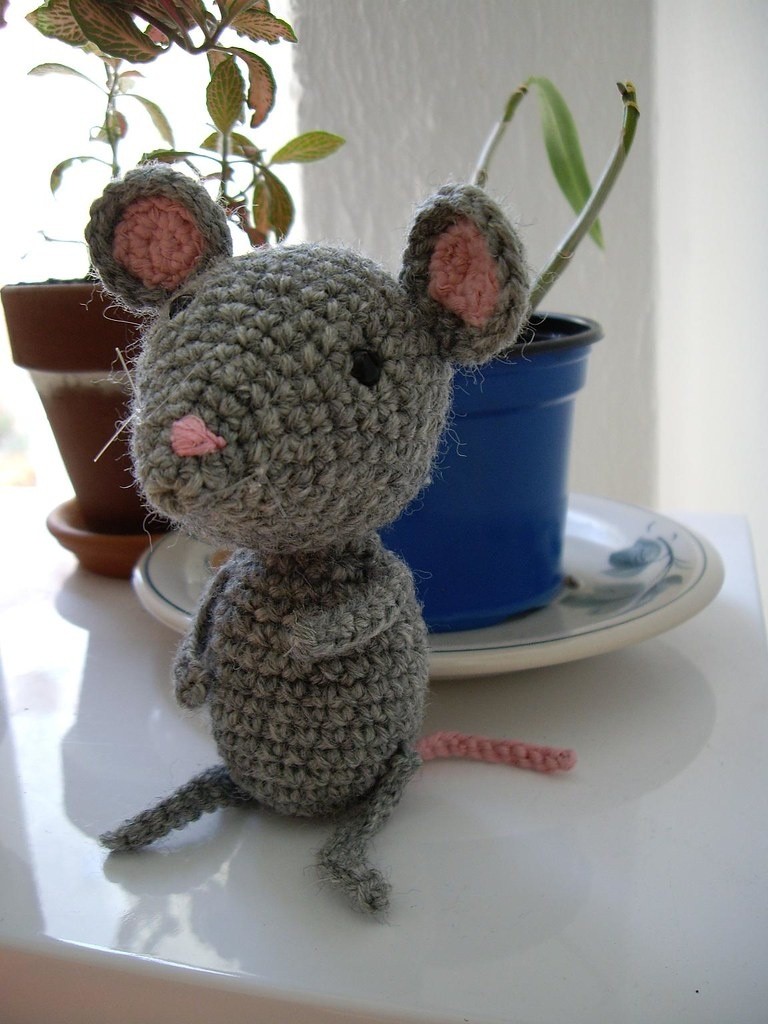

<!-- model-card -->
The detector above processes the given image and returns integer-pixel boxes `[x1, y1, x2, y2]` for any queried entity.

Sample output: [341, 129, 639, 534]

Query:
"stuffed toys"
[85, 162, 578, 926]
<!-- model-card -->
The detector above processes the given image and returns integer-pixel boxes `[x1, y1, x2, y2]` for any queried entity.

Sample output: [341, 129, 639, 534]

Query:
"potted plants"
[375, 77, 639, 634]
[0, 1, 348, 534]
